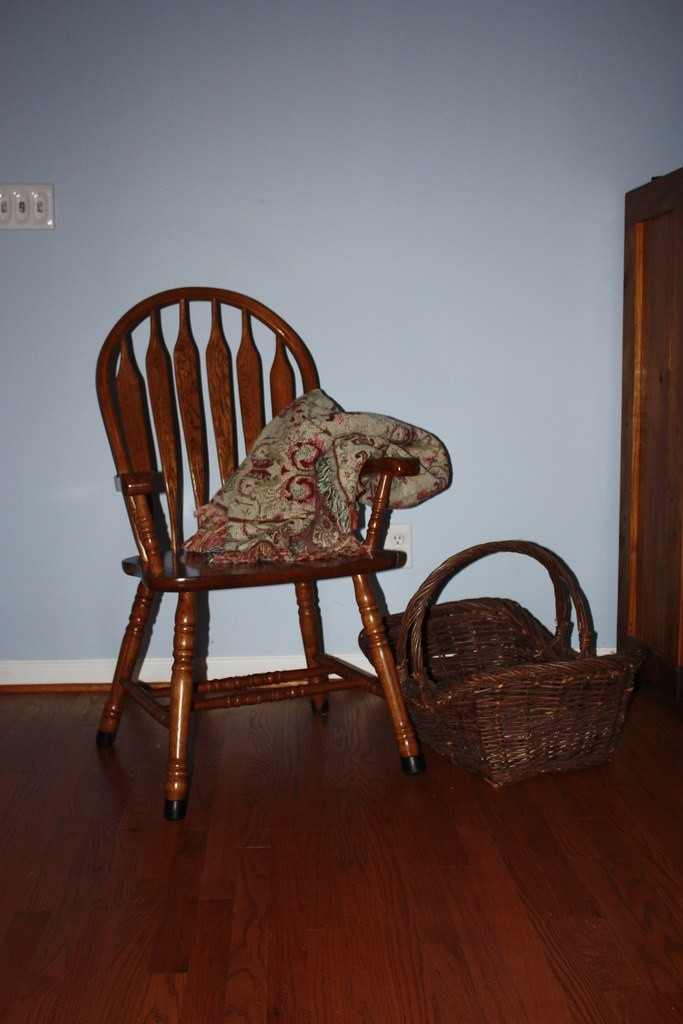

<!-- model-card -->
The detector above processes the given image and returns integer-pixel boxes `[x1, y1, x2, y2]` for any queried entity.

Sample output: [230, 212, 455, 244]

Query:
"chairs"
[96, 287, 424, 824]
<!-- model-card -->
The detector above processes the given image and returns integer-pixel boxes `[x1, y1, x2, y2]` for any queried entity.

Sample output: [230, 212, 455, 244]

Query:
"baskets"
[358, 540, 643, 787]
